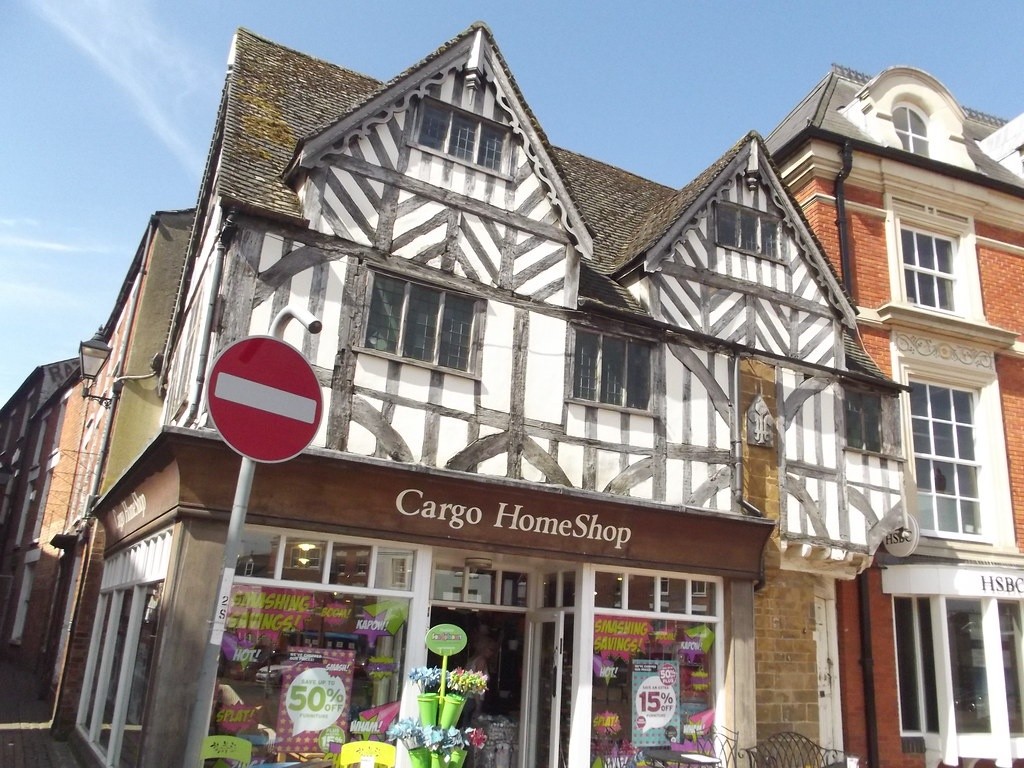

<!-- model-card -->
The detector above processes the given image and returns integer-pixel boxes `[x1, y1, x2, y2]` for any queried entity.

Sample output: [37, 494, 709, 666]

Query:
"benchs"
[739, 732, 865, 768]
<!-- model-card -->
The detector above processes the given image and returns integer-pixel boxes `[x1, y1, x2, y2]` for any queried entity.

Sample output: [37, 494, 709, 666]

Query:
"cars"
[253, 651, 324, 686]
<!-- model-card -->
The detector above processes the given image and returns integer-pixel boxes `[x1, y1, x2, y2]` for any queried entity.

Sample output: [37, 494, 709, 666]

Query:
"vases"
[431, 753, 449, 768]
[440, 693, 467, 730]
[448, 749, 468, 768]
[417, 693, 439, 728]
[407, 748, 431, 768]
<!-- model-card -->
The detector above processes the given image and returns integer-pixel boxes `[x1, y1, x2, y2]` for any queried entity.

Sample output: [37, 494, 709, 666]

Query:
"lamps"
[79, 324, 114, 409]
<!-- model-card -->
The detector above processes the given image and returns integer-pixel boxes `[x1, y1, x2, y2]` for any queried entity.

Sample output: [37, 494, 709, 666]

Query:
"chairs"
[340, 740, 396, 768]
[694, 725, 740, 768]
[199, 735, 252, 768]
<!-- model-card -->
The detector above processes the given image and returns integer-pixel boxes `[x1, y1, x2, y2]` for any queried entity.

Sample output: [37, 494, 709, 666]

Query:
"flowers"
[420, 723, 464, 763]
[461, 726, 488, 750]
[451, 666, 490, 698]
[385, 717, 424, 750]
[409, 665, 450, 693]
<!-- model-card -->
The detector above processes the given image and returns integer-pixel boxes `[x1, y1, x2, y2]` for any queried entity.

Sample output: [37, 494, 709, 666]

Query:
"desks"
[644, 750, 723, 768]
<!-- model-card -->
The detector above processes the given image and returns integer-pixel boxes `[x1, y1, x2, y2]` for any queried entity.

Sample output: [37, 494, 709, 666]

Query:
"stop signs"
[204, 333, 325, 464]
